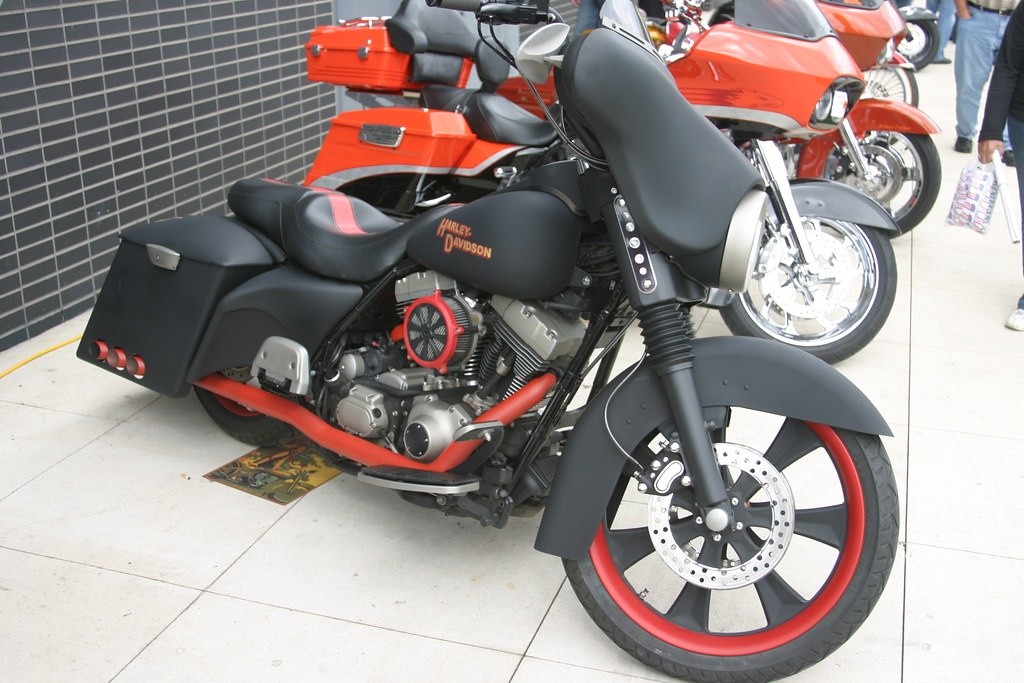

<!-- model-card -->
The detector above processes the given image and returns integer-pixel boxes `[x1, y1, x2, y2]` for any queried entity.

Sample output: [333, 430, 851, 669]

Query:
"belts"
[966, 0, 1016, 17]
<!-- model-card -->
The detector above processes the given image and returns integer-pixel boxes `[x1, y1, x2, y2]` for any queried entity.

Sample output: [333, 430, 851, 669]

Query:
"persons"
[571, 0, 605, 42]
[978, 1, 1024, 332]
[926, 0, 957, 63]
[954, 0, 1019, 168]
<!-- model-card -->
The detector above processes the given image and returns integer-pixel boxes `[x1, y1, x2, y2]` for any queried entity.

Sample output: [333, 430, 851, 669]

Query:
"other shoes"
[929, 57, 951, 64]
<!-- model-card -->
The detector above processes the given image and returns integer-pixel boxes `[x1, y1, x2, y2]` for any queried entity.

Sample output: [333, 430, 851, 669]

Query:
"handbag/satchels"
[945, 161, 997, 234]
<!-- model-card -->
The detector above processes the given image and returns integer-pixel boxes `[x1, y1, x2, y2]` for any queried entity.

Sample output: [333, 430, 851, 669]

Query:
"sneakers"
[1003, 148, 1018, 167]
[1006, 306, 1024, 330]
[953, 137, 973, 152]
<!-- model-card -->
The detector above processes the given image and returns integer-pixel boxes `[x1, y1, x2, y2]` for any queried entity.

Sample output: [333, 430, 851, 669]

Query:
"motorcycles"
[82, 2, 945, 682]
